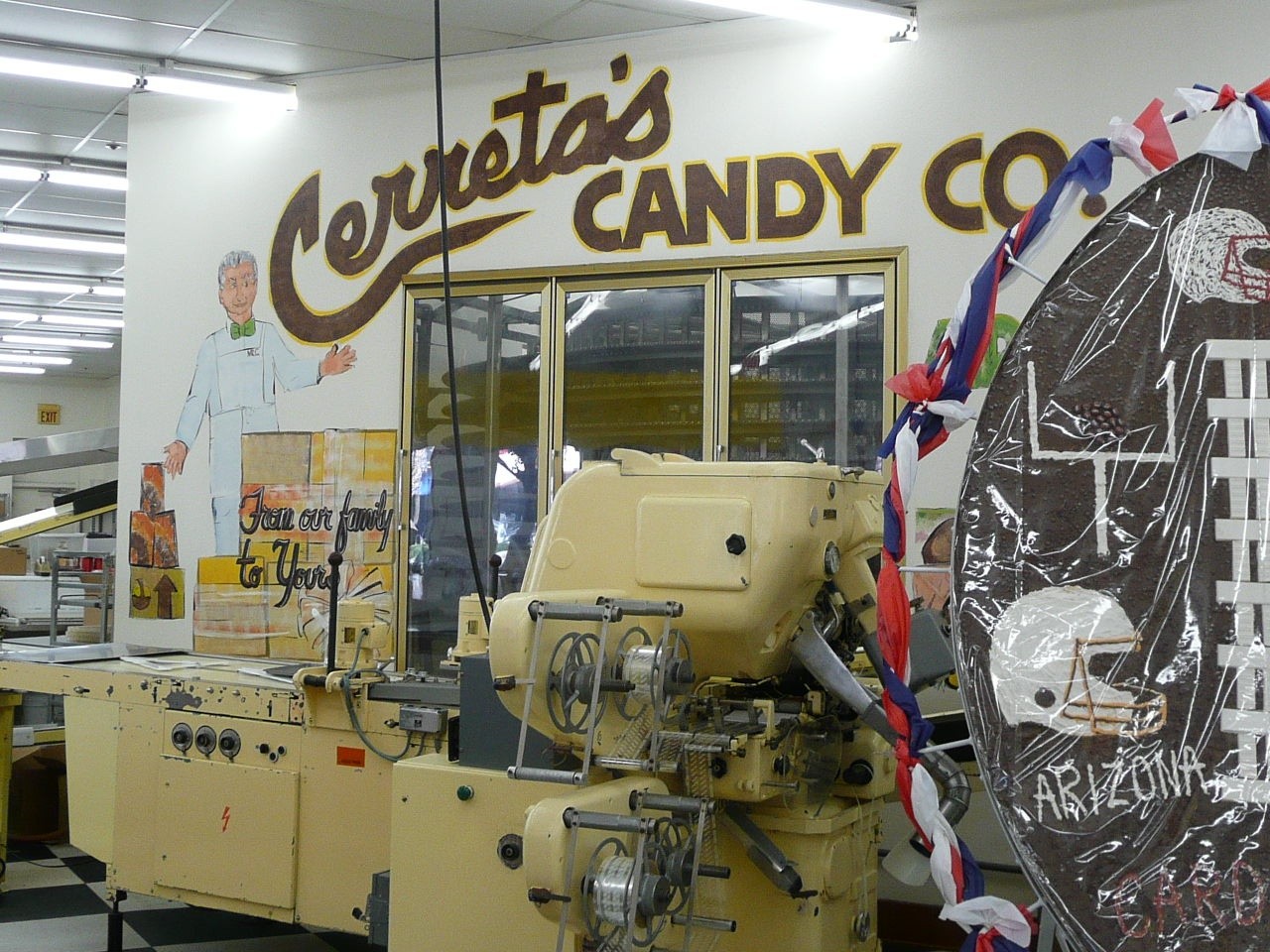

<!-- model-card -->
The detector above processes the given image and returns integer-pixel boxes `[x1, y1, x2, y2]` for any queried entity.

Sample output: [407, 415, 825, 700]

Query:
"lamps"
[0, 39, 298, 115]
[689, 1, 920, 43]
[0, 158, 127, 191]
[1, 272, 125, 297]
[1, 223, 126, 254]
